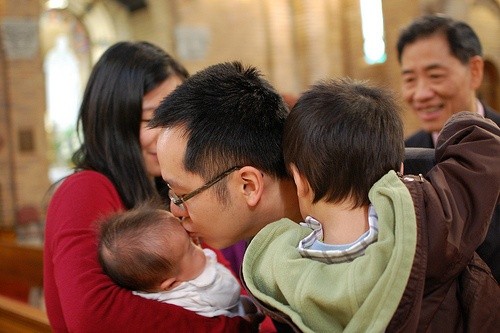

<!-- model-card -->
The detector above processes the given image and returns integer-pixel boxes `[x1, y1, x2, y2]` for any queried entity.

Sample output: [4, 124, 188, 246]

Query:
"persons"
[97, 197, 258, 318]
[43, 42, 277, 333]
[149, 58, 500, 333]
[395, 14, 500, 148]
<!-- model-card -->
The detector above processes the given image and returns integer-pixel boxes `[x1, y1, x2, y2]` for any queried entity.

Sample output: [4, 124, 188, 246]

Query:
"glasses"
[168, 167, 264, 211]
